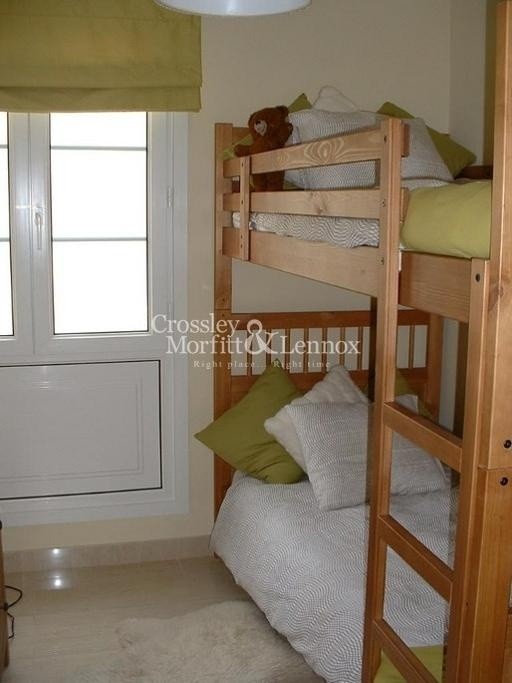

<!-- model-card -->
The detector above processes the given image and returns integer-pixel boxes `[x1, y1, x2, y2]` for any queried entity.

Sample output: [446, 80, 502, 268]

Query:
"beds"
[212, 0, 512, 683]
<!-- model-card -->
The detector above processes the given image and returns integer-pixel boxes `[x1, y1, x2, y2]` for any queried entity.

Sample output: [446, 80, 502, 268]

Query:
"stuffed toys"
[234, 104, 294, 192]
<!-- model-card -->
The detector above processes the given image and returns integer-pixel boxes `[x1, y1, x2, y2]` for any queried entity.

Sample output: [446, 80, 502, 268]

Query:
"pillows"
[262, 362, 370, 477]
[371, 644, 446, 683]
[279, 84, 370, 191]
[216, 93, 312, 192]
[193, 358, 309, 485]
[372, 101, 477, 181]
[361, 365, 436, 426]
[284, 392, 448, 513]
[282, 109, 456, 190]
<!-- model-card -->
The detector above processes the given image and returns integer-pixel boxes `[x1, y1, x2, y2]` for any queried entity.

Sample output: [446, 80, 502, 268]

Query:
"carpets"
[109, 594, 312, 683]
